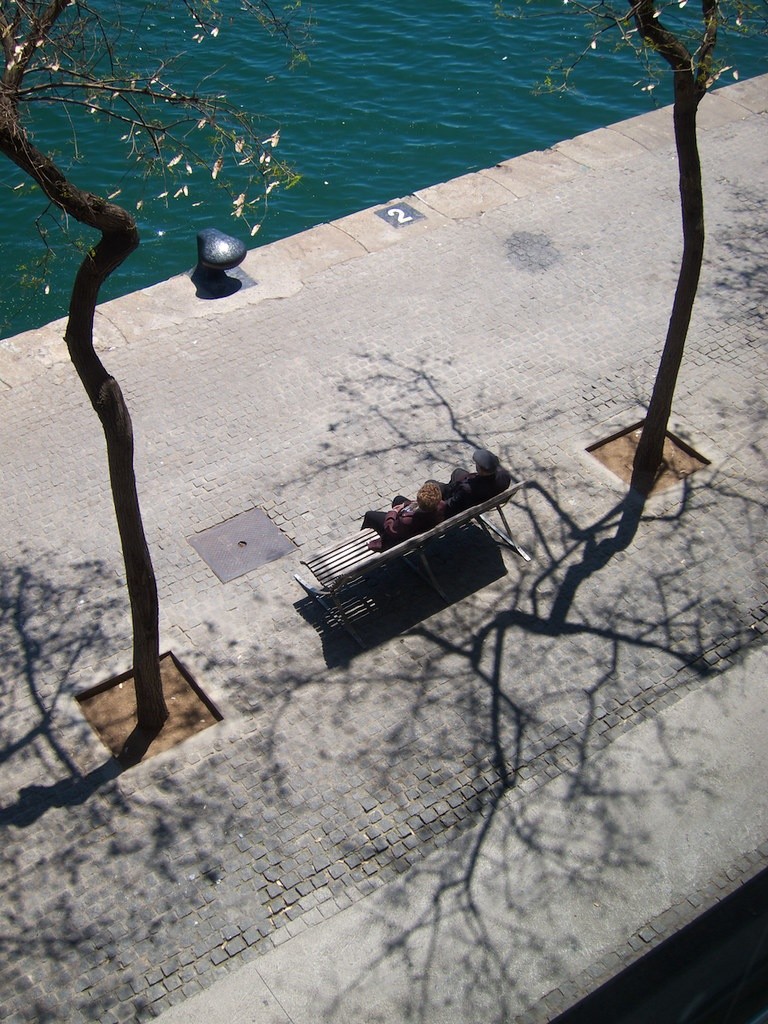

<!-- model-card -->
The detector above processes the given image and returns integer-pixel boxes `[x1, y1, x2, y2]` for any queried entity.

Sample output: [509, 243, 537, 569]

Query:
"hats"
[473, 450, 499, 474]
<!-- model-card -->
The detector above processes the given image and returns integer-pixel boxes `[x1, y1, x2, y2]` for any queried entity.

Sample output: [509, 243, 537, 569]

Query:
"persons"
[424, 448, 510, 515]
[360, 482, 448, 552]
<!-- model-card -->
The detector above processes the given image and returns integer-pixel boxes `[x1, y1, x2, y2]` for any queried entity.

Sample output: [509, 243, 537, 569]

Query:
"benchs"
[288, 478, 534, 648]
